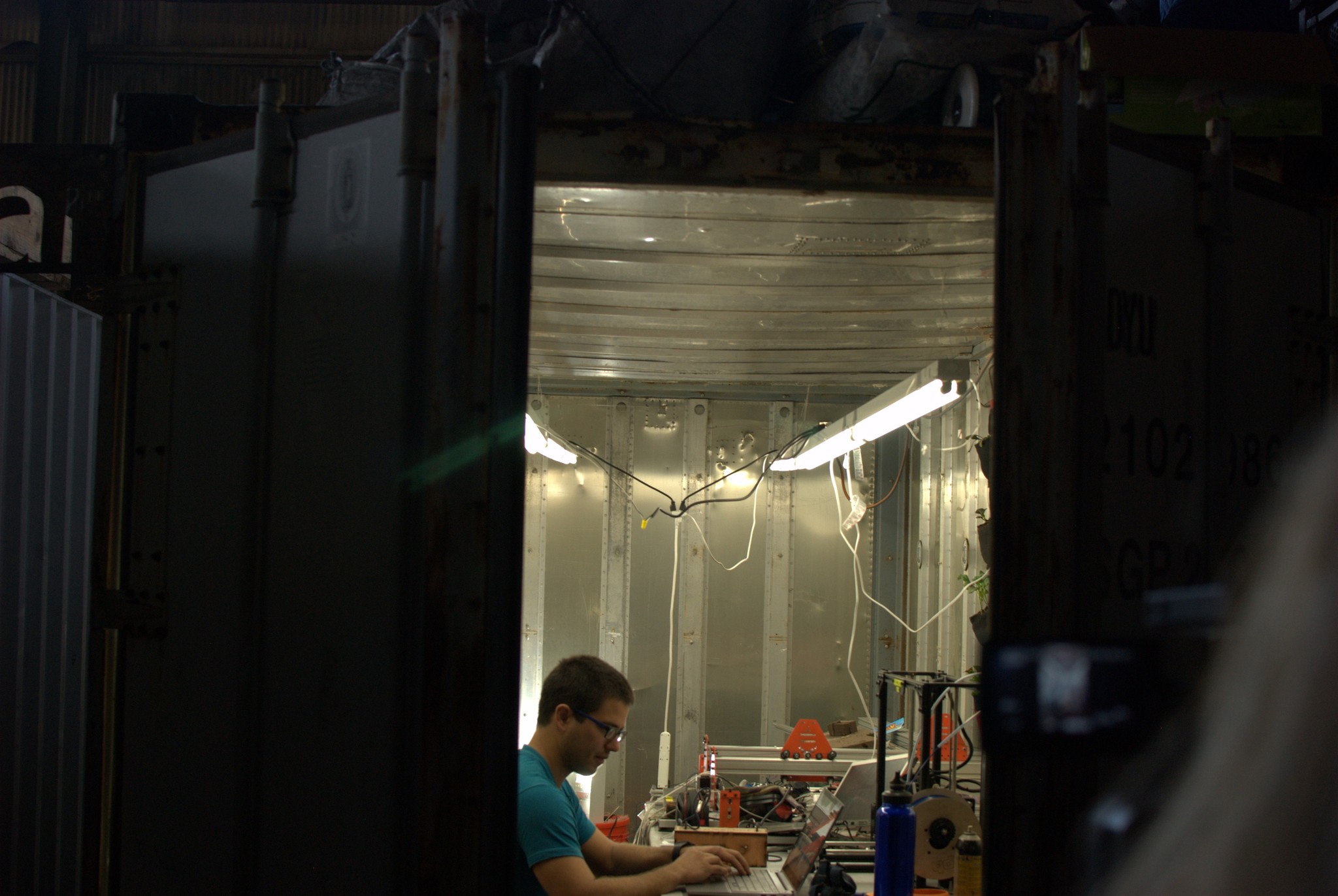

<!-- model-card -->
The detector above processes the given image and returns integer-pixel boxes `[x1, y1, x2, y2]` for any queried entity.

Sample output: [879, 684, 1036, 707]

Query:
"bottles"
[874, 771, 916, 896]
[954, 825, 982, 896]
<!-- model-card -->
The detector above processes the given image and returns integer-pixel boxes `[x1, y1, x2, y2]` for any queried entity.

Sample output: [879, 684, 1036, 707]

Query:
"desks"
[650, 808, 878, 896]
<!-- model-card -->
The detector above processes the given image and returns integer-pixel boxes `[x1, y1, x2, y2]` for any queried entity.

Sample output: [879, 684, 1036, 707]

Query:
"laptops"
[830, 751, 911, 827]
[685, 787, 845, 896]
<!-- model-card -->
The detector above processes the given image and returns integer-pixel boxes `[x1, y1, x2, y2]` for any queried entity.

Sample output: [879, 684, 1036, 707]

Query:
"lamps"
[768, 353, 973, 477]
[524, 398, 579, 466]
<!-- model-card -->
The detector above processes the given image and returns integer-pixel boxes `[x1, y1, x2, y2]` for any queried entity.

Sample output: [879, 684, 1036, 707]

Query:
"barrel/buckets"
[594, 815, 630, 843]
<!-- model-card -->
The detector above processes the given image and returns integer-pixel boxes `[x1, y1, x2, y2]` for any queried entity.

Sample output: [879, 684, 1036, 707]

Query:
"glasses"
[569, 709, 628, 743]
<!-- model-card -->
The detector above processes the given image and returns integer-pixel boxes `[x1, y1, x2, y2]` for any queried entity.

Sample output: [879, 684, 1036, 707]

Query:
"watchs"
[672, 841, 695, 861]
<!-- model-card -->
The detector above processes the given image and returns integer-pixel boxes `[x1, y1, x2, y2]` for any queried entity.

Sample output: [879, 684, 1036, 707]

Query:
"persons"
[1083, 389, 1338, 896]
[519, 655, 752, 896]
